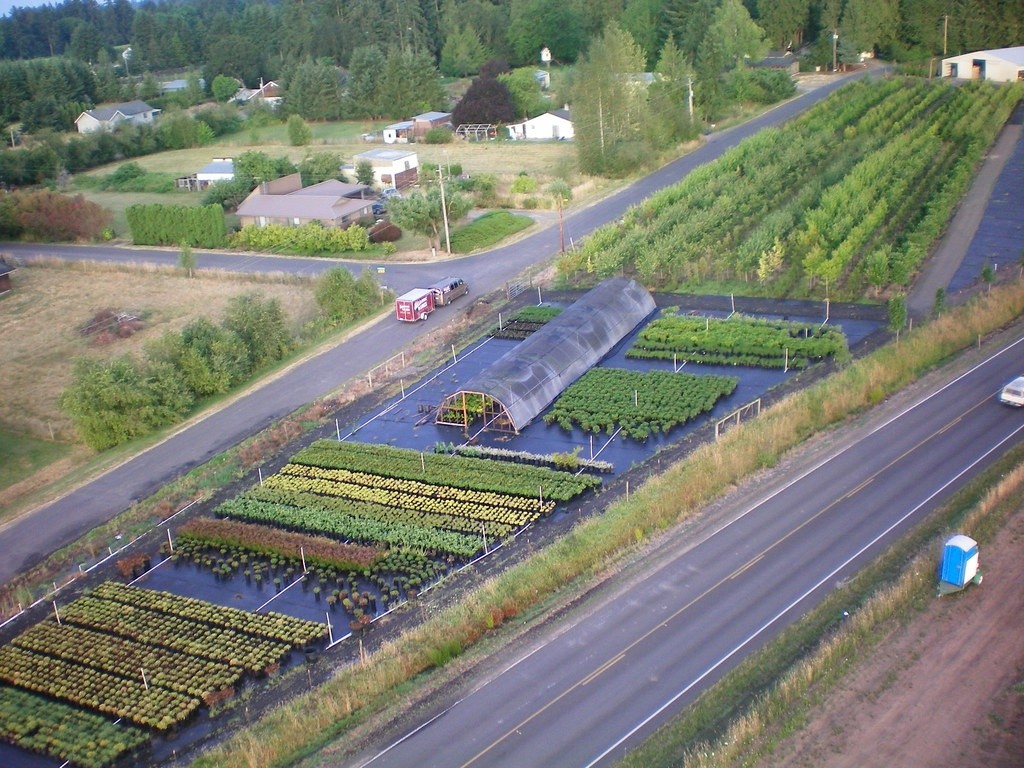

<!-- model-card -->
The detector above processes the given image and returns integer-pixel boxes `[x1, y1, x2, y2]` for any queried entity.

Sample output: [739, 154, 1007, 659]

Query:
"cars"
[1000, 377, 1024, 408]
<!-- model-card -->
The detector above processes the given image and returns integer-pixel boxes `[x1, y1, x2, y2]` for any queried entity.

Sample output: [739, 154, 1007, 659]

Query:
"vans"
[427, 277, 469, 307]
